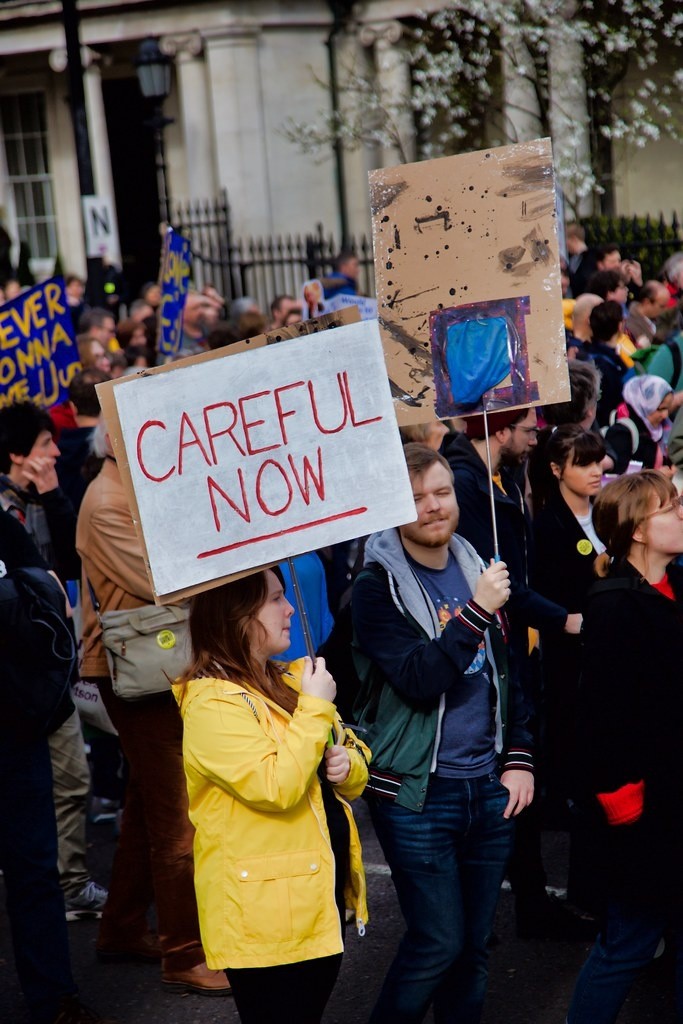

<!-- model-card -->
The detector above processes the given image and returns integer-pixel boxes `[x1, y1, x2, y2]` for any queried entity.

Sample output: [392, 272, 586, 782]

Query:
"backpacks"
[313, 567, 388, 725]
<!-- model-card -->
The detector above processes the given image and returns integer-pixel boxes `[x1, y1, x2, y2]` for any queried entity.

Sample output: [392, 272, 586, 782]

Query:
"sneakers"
[64, 880, 110, 921]
[161, 962, 232, 995]
[49, 994, 122, 1024]
[121, 940, 162, 962]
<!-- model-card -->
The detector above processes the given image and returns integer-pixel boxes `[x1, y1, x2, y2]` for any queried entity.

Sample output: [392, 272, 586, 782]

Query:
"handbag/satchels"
[98, 605, 194, 699]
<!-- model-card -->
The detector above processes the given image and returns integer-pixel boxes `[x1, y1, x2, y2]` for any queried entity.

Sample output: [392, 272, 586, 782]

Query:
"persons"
[166, 566, 372, 1023]
[1, 222, 683, 1024]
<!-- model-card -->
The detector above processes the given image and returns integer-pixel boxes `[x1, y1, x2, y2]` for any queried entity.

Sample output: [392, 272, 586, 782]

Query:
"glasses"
[510, 425, 539, 439]
[639, 497, 682, 524]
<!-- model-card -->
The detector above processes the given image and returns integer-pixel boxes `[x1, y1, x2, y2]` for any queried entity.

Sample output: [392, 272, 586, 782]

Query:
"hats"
[463, 408, 529, 435]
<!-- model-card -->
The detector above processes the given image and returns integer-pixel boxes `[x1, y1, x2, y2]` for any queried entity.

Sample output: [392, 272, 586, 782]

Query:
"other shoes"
[517, 892, 593, 942]
